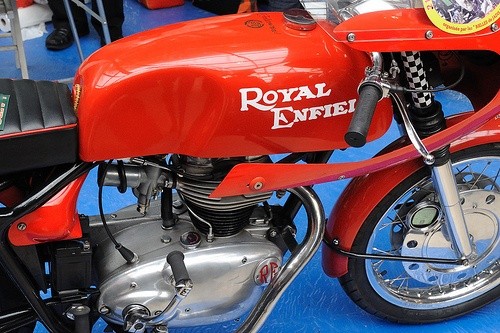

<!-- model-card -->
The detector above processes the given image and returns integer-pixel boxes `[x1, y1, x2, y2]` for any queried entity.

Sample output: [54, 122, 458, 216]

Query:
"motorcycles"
[0, 0, 500, 333]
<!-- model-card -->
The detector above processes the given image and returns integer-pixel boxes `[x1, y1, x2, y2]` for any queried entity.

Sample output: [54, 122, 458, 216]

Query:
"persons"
[46, 0, 124, 51]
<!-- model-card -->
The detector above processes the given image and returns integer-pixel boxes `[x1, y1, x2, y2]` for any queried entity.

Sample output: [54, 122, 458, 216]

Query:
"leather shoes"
[100, 37, 122, 47]
[46, 28, 74, 51]
